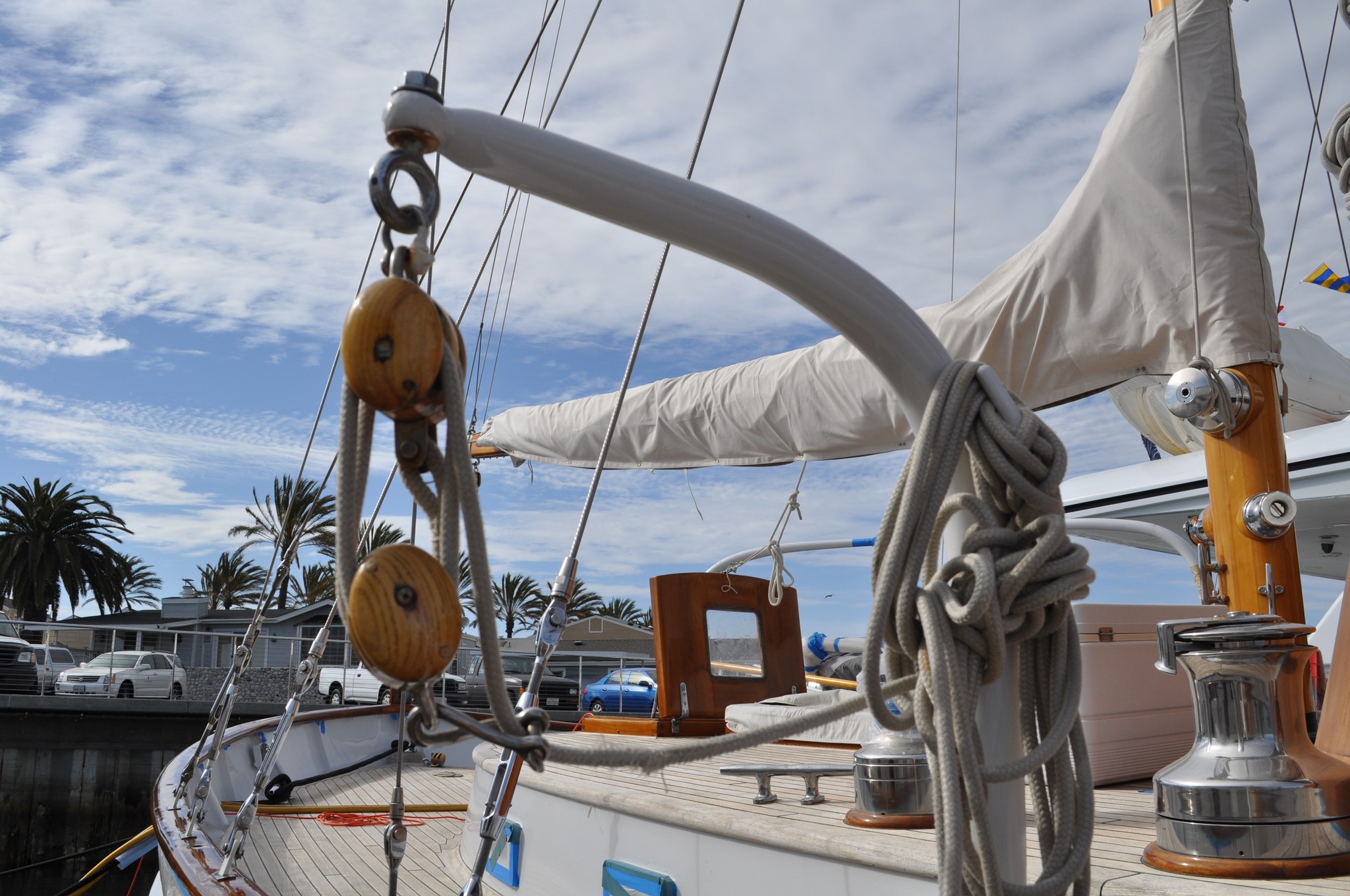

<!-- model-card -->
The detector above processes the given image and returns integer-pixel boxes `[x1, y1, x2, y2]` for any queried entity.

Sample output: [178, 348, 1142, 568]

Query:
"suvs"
[29, 643, 78, 694]
[53, 648, 188, 702]
[0, 610, 38, 695]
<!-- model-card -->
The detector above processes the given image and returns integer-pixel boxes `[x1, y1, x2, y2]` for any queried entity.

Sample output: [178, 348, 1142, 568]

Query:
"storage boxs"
[1071, 603, 1229, 786]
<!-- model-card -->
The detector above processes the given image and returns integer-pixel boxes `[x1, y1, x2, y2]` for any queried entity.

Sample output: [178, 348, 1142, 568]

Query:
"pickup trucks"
[454, 652, 581, 712]
[318, 658, 469, 710]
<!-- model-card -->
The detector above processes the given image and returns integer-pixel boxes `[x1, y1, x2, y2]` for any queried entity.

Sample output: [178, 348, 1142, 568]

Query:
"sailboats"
[148, 2, 1350, 896]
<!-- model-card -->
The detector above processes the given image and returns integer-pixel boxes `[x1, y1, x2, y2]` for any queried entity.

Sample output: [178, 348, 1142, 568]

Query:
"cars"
[581, 666, 659, 714]
